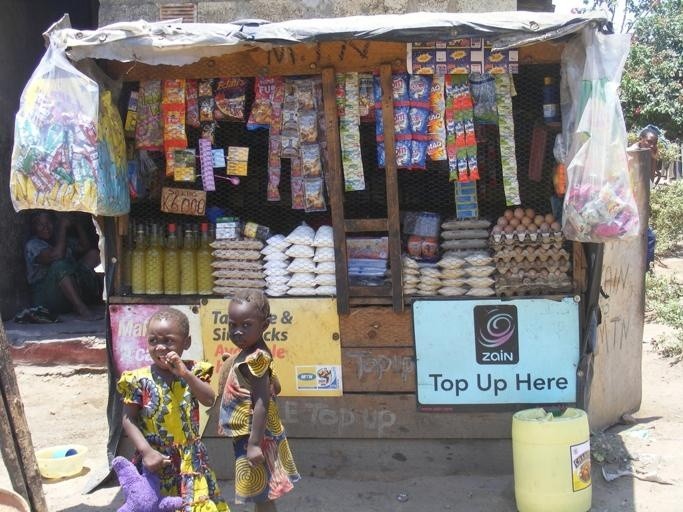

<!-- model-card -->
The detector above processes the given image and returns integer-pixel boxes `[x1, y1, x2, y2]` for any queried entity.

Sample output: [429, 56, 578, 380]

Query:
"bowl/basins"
[34, 444, 86, 478]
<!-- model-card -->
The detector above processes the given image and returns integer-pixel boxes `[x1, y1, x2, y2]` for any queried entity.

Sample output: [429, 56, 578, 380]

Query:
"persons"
[116, 308, 230, 512]
[628, 129, 658, 270]
[23, 211, 104, 321]
[217, 288, 303, 512]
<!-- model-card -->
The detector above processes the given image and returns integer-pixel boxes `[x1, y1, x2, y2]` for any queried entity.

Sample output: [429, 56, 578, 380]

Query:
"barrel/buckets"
[512, 407, 592, 512]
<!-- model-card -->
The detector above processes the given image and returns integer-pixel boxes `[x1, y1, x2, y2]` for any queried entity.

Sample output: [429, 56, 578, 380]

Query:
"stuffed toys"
[111, 456, 187, 512]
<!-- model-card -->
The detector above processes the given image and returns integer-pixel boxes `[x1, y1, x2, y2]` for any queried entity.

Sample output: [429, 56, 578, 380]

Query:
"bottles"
[130, 224, 146, 294]
[196, 223, 216, 295]
[165, 223, 180, 295]
[160, 226, 165, 246]
[193, 224, 201, 247]
[542, 76, 556, 123]
[181, 223, 198, 295]
[145, 225, 150, 245]
[208, 224, 214, 237]
[146, 224, 164, 294]
[122, 223, 133, 287]
[177, 226, 184, 248]
